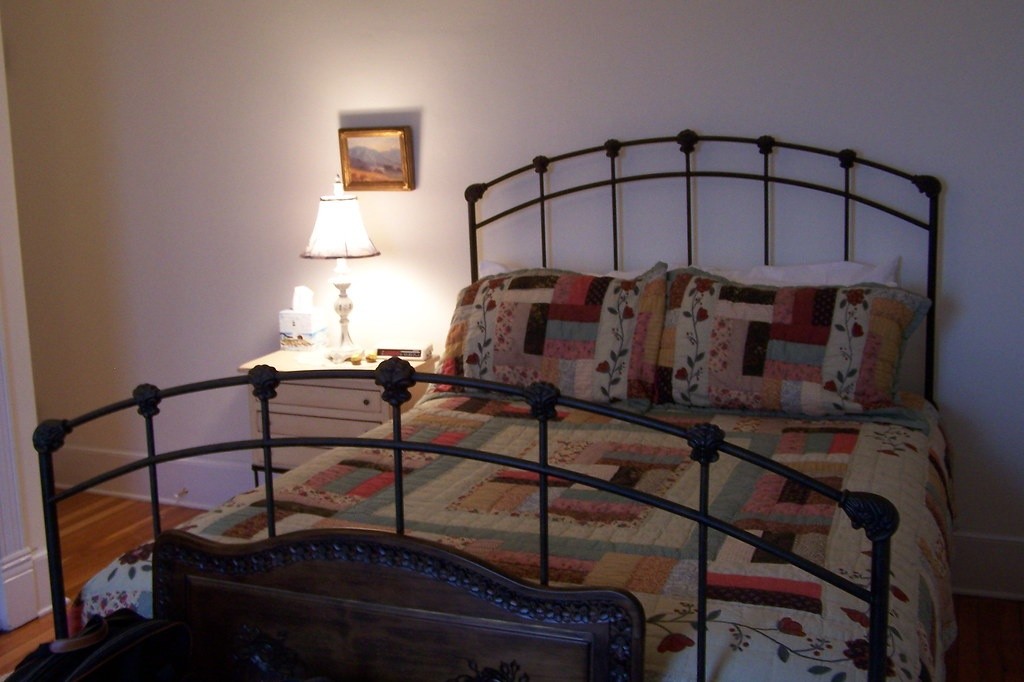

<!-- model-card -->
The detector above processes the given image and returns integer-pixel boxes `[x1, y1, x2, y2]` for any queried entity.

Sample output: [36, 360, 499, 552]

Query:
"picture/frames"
[338, 127, 415, 192]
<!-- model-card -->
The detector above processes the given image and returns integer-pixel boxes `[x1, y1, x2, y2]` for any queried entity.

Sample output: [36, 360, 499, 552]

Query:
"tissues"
[278, 285, 330, 351]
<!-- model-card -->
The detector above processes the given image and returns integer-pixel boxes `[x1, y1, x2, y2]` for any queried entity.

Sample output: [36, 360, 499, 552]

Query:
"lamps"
[299, 173, 380, 365]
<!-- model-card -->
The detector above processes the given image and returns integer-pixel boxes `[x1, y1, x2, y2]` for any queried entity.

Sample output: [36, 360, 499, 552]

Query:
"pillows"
[432, 259, 670, 404]
[672, 275, 932, 420]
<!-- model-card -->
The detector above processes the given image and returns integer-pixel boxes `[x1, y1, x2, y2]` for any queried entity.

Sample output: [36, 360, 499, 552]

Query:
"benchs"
[4, 527, 646, 682]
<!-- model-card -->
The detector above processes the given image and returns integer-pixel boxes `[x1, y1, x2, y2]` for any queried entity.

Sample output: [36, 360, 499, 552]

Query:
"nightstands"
[237, 346, 441, 488]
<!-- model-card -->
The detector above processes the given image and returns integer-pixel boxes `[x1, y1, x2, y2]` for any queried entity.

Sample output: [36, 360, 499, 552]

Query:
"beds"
[31, 131, 960, 682]
